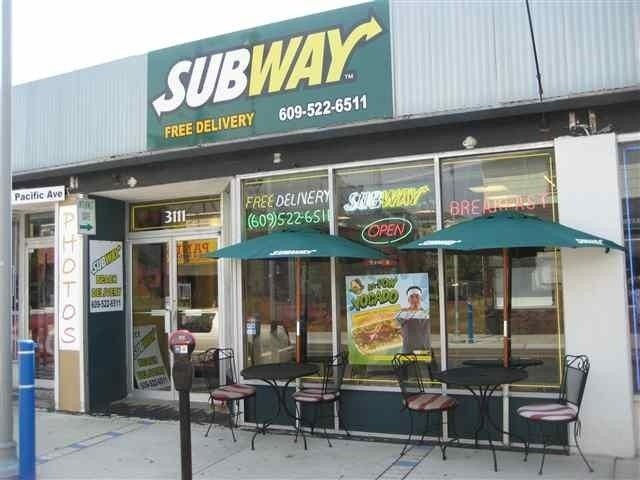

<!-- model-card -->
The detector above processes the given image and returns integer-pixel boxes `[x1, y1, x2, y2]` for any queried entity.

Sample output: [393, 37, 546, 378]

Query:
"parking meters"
[246, 313, 263, 368]
[167, 327, 197, 480]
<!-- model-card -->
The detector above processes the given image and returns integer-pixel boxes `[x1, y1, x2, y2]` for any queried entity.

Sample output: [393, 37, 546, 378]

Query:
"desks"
[436, 367, 529, 471]
[241, 363, 319, 449]
[464, 359, 544, 406]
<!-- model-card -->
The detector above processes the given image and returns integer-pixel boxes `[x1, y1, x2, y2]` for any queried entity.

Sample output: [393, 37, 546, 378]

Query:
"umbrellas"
[395, 206, 629, 367]
[203, 223, 393, 364]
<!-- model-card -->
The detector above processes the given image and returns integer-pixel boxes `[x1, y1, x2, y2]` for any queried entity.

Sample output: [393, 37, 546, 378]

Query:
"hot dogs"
[351, 305, 402, 354]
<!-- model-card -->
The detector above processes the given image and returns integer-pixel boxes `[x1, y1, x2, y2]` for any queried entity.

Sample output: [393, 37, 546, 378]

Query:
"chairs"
[392, 352, 459, 460]
[292, 351, 351, 447]
[200, 348, 261, 442]
[517, 354, 592, 473]
[427, 353, 442, 387]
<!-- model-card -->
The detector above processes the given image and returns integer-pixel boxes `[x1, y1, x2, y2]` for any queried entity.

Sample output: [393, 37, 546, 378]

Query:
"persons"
[394, 286, 430, 354]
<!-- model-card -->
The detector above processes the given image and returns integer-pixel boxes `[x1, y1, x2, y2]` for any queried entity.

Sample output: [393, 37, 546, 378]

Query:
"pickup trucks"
[177, 306, 295, 374]
